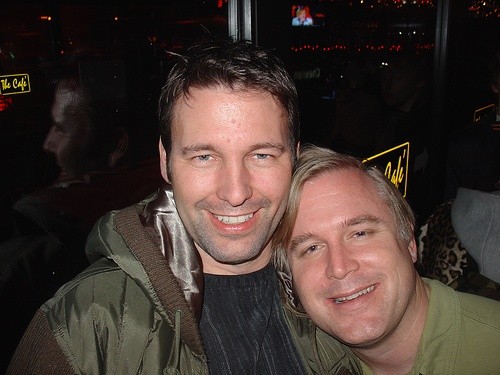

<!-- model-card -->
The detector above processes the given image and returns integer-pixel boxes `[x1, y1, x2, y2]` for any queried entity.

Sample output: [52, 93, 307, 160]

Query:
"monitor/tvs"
[288, 0, 327, 32]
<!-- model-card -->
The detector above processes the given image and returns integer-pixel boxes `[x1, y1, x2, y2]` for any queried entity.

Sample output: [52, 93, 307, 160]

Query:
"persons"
[271, 143, 500, 375]
[414, 122, 500, 302]
[5, 35, 364, 375]
[292, 8, 312, 26]
[0, 71, 150, 351]
[326, 56, 413, 142]
